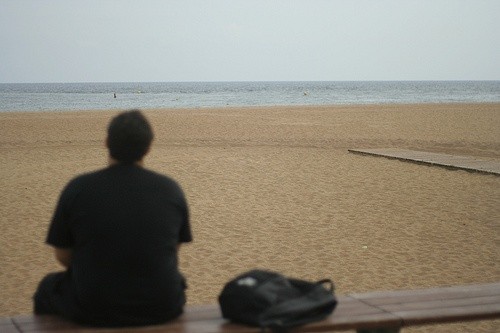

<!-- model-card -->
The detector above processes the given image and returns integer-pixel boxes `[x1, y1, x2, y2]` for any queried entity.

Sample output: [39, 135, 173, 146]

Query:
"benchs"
[1, 281, 404, 333]
[347, 277, 500, 333]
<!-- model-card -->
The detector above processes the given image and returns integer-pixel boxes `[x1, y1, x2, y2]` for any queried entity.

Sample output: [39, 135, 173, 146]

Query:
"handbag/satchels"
[217, 266, 338, 333]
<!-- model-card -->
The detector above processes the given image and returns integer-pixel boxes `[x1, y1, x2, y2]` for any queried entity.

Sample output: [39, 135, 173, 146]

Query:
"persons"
[30, 108, 193, 328]
